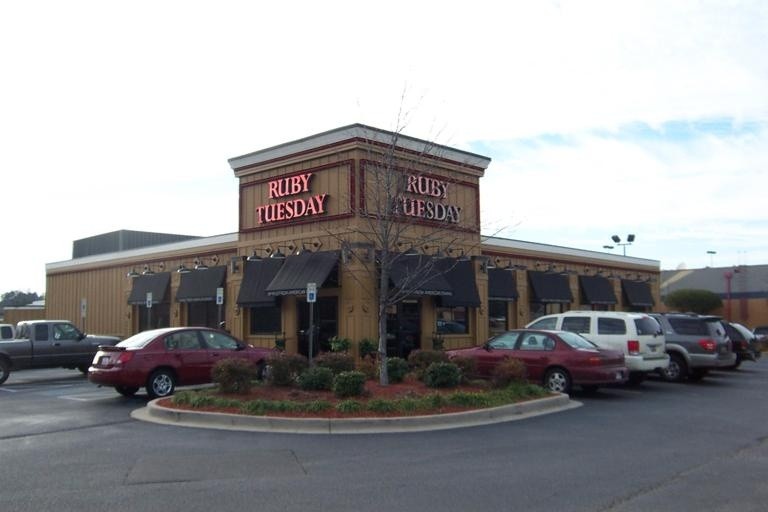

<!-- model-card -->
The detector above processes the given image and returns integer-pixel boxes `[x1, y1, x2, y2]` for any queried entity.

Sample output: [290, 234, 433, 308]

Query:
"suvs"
[647, 312, 737, 384]
[492, 310, 671, 387]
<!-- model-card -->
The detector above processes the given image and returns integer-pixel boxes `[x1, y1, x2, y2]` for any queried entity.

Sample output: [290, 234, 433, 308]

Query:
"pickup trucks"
[0, 318, 123, 385]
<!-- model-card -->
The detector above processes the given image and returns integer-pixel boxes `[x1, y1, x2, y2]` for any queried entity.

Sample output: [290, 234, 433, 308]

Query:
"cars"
[442, 326, 629, 395]
[721, 321, 762, 369]
[302, 314, 467, 361]
[753, 326, 768, 352]
[0, 323, 16, 341]
[88, 324, 286, 397]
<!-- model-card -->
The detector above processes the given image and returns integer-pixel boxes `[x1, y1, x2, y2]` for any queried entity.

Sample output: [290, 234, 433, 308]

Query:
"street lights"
[723, 269, 733, 321]
[610, 233, 636, 255]
[705, 249, 716, 269]
